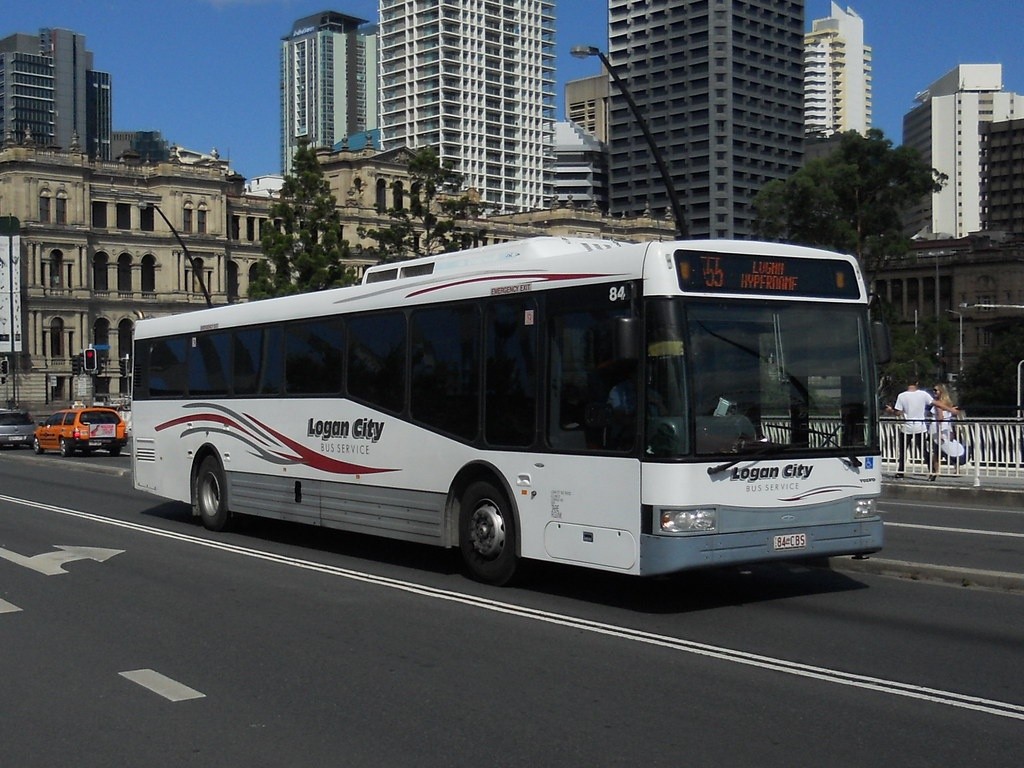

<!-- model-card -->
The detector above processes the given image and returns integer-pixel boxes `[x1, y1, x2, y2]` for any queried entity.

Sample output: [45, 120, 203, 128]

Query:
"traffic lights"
[72, 356, 79, 376]
[119, 360, 127, 377]
[84, 349, 96, 369]
[2, 360, 9, 374]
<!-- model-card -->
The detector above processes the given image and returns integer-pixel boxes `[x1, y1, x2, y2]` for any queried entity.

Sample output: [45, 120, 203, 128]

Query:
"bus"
[128, 235, 889, 587]
[93, 393, 133, 430]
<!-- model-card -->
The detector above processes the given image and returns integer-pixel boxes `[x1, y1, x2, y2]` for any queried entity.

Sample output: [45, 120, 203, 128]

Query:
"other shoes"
[930, 472, 939, 481]
[895, 472, 904, 478]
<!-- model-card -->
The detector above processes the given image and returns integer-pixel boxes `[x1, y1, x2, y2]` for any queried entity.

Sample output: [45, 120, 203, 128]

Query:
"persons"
[926, 382, 970, 473]
[884, 376, 960, 481]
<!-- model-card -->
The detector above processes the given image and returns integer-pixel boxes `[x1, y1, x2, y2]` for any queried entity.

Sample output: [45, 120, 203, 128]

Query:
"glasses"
[933, 389, 938, 391]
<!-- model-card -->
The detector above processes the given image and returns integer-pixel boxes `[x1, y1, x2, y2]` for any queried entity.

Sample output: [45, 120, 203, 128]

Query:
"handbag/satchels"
[941, 439, 964, 456]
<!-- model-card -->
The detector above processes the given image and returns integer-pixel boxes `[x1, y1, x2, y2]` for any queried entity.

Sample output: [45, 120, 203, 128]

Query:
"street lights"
[136, 201, 214, 309]
[569, 45, 691, 240]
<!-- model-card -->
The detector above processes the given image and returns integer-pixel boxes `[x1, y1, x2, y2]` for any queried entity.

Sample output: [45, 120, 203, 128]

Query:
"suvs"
[33, 401, 128, 458]
[0, 410, 35, 449]
